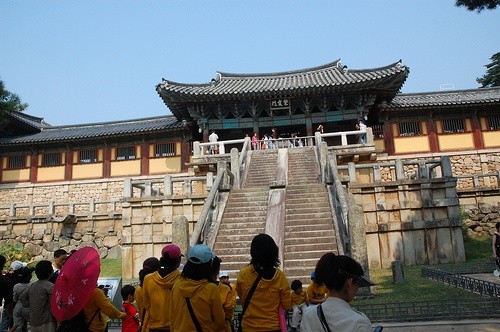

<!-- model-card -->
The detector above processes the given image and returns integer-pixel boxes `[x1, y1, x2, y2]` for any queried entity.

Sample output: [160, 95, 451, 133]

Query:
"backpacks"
[59, 310, 88, 332]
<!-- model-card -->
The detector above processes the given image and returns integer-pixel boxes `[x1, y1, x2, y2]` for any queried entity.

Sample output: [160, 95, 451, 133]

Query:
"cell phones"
[374, 326, 384, 332]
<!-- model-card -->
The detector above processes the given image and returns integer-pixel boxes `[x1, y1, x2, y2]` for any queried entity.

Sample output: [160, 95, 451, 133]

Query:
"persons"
[354, 120, 369, 145]
[135, 256, 163, 325]
[316, 124, 325, 142]
[291, 253, 378, 332]
[210, 255, 233, 323]
[0, 255, 8, 308]
[235, 233, 292, 332]
[0, 261, 29, 332]
[242, 128, 278, 150]
[82, 284, 127, 332]
[120, 284, 140, 332]
[491, 221, 500, 281]
[50, 248, 70, 284]
[27, 260, 56, 332]
[143, 244, 185, 332]
[219, 271, 237, 323]
[12, 267, 33, 332]
[169, 244, 233, 332]
[208, 130, 218, 156]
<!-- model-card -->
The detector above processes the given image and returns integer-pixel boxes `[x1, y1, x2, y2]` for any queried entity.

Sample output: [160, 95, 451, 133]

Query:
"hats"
[9, 261, 28, 271]
[177, 264, 184, 272]
[187, 244, 216, 264]
[322, 255, 375, 287]
[161, 244, 185, 259]
[218, 271, 229, 278]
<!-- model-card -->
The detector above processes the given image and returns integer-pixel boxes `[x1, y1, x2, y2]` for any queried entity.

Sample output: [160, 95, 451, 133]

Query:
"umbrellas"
[51, 246, 100, 321]
